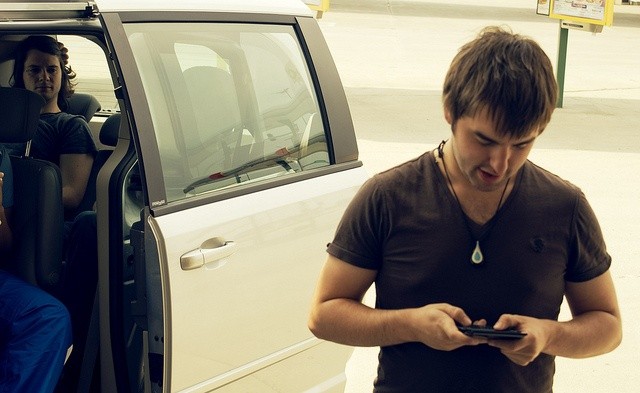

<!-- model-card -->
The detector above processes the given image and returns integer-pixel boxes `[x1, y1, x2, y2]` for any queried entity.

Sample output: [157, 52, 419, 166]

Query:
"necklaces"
[436, 140, 511, 268]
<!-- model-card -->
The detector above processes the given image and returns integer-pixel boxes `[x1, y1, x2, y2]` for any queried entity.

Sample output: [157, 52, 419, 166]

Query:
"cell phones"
[456, 324, 527, 342]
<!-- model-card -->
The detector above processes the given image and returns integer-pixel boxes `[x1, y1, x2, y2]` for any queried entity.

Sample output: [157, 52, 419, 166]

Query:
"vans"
[0, 0, 369, 393]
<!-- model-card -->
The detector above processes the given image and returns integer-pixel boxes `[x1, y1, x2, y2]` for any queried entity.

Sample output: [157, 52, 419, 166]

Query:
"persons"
[1, 145, 75, 393]
[306, 26, 624, 393]
[8, 35, 97, 208]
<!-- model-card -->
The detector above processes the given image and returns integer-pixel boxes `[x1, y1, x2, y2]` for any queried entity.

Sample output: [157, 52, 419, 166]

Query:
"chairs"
[65, 92, 102, 124]
[0, 85, 63, 287]
[93, 113, 121, 185]
